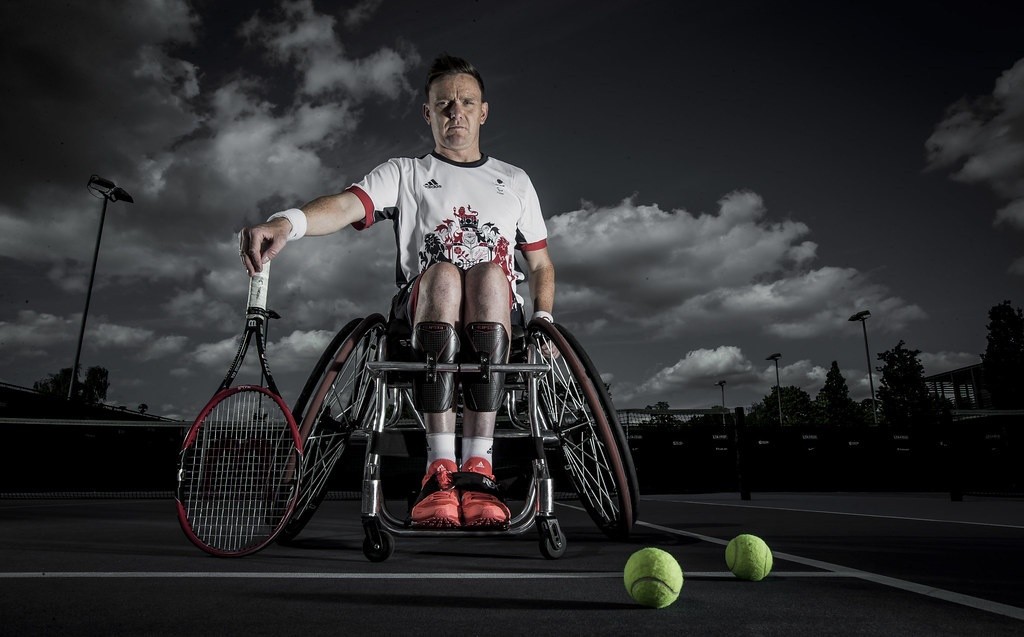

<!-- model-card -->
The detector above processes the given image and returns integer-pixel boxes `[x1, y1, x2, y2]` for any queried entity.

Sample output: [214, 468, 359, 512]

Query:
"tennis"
[622, 546, 684, 609]
[725, 533, 773, 582]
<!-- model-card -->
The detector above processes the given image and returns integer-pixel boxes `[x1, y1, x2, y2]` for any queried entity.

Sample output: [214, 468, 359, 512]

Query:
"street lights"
[766, 352, 784, 429]
[714, 379, 726, 435]
[66, 171, 135, 403]
[847, 309, 878, 425]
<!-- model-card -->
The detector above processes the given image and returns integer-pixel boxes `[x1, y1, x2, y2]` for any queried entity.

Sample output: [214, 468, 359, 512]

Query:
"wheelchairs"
[261, 292, 643, 564]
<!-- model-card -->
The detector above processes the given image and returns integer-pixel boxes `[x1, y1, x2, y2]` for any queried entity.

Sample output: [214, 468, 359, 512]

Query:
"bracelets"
[531, 311, 553, 323]
[266, 208, 307, 241]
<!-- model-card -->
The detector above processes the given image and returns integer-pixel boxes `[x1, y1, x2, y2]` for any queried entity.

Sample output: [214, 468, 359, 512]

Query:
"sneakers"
[411, 458, 462, 528]
[461, 457, 511, 526]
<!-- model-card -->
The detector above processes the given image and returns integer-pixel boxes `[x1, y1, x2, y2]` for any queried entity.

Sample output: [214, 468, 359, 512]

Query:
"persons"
[236, 58, 559, 528]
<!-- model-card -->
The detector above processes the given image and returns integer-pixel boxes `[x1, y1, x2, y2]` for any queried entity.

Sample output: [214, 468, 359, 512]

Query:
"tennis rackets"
[174, 237, 307, 559]
